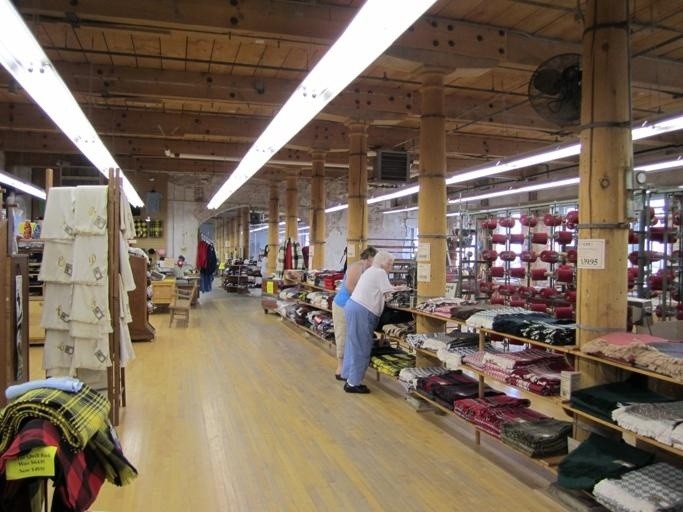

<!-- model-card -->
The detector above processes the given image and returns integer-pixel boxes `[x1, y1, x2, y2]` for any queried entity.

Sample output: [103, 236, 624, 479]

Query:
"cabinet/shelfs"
[0, 166, 134, 512]
[221, 185, 682, 512]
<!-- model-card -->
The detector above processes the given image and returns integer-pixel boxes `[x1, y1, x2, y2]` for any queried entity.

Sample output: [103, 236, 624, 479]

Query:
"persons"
[340, 251, 395, 393]
[331, 247, 378, 379]
[173, 256, 192, 279]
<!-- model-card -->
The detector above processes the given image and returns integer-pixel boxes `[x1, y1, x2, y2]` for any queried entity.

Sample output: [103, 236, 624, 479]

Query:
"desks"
[151, 275, 176, 313]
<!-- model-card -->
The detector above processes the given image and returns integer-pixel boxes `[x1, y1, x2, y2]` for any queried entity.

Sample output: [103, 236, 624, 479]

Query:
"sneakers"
[334, 375, 369, 394]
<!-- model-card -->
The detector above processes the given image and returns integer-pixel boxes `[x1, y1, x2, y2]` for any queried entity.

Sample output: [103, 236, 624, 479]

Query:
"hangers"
[150, 186, 156, 193]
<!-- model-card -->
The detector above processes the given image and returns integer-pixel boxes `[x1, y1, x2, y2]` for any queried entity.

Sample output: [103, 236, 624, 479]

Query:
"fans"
[530, 53, 588, 126]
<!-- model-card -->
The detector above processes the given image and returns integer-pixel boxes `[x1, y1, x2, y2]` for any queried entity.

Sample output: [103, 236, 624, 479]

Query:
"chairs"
[168, 280, 197, 324]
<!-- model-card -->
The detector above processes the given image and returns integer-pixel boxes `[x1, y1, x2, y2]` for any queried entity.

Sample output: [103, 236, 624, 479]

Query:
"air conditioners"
[374, 149, 408, 182]
